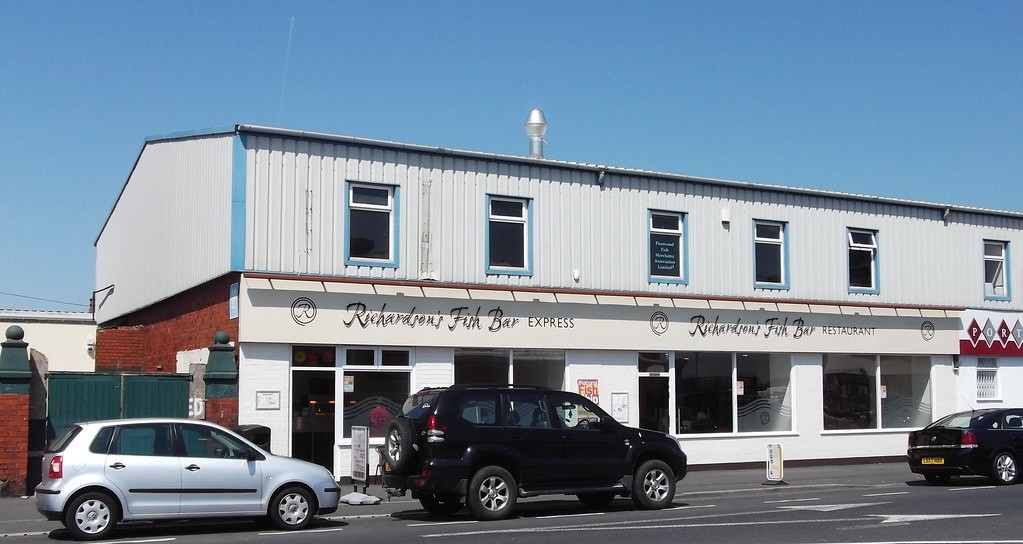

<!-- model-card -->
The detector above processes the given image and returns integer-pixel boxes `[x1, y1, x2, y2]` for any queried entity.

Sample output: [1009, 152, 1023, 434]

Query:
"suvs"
[378, 382, 689, 521]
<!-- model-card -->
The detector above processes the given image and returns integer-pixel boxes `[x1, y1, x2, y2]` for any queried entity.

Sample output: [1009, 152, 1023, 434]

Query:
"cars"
[34, 416, 342, 542]
[907, 407, 1023, 486]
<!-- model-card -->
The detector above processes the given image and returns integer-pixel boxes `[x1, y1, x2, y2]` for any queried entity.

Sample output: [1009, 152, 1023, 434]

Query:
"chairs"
[1009, 417, 1022, 428]
[509, 411, 523, 428]
[530, 408, 548, 428]
